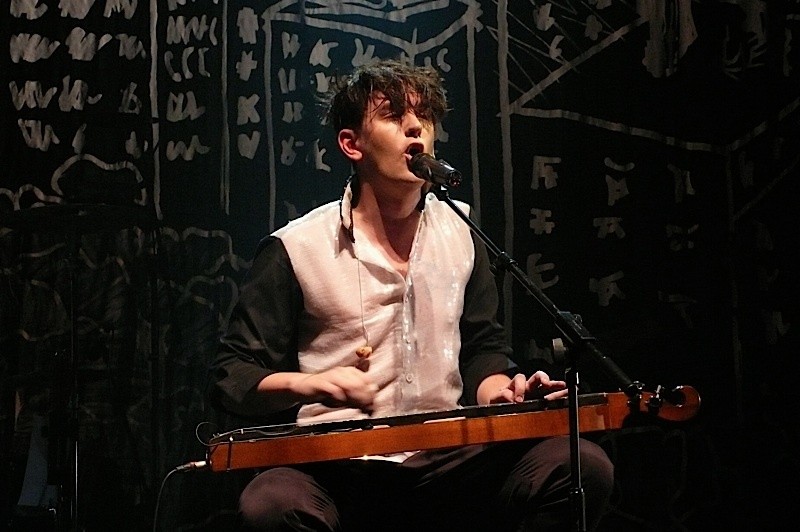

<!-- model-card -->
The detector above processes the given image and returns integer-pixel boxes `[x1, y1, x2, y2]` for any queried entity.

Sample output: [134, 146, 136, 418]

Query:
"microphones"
[410, 152, 462, 189]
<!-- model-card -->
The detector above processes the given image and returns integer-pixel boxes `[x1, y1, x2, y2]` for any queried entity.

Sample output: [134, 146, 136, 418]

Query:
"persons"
[197, 63, 615, 532]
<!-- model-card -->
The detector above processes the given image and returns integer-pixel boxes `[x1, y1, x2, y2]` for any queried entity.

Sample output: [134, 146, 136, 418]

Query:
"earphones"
[356, 346, 372, 358]
